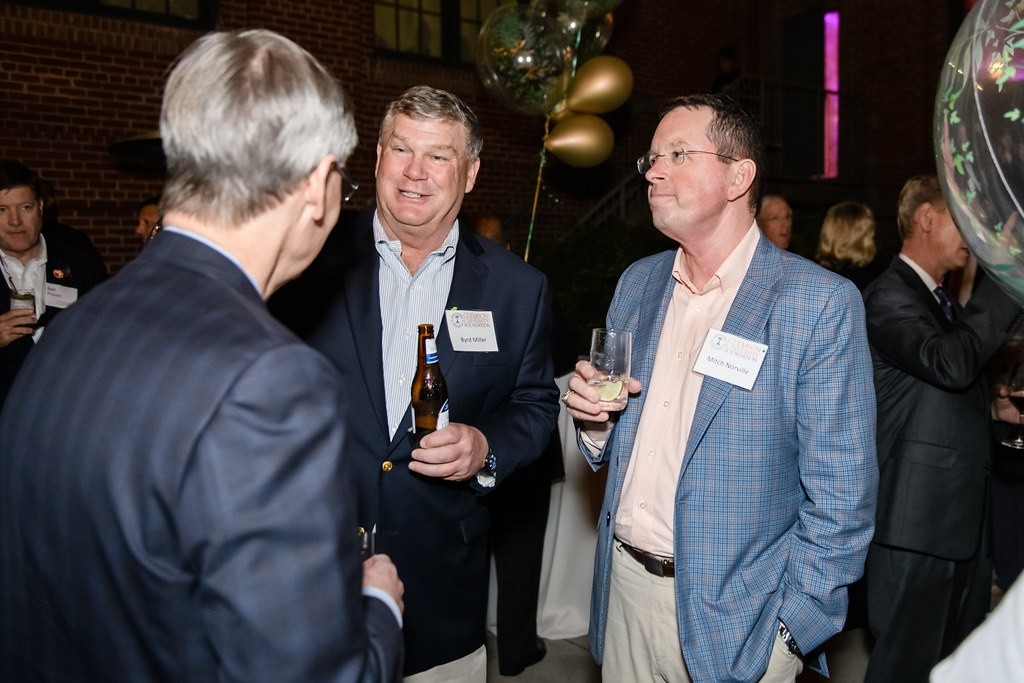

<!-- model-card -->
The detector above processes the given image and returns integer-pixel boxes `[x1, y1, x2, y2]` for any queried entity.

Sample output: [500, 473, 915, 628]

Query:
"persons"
[459, 210, 566, 676]
[813, 202, 882, 289]
[755, 193, 793, 249]
[265, 86, 560, 676]
[865, 172, 1024, 683]
[564, 96, 878, 683]
[0, 26, 405, 683]
[0, 158, 110, 413]
[136, 196, 163, 246]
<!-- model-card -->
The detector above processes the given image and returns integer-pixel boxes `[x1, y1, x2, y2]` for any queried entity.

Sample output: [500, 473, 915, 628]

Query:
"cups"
[588, 329, 632, 412]
[355, 522, 375, 570]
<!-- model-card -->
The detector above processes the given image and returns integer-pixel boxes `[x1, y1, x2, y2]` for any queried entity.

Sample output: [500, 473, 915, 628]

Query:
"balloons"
[566, 56, 634, 114]
[475, 0, 613, 116]
[545, 114, 614, 168]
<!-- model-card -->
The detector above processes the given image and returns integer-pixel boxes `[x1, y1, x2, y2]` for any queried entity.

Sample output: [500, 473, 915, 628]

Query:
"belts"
[613, 535, 675, 578]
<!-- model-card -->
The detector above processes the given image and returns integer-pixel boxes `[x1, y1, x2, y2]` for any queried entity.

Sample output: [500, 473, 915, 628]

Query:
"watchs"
[779, 626, 801, 655]
[480, 449, 496, 474]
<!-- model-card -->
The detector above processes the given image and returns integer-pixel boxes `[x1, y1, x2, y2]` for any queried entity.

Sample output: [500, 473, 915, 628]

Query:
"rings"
[562, 389, 575, 403]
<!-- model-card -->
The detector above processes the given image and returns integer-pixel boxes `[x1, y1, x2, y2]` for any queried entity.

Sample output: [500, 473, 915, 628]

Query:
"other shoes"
[500, 638, 547, 676]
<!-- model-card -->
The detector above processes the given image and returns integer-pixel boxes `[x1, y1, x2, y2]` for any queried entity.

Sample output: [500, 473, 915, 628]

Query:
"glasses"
[307, 161, 360, 203]
[636, 148, 739, 175]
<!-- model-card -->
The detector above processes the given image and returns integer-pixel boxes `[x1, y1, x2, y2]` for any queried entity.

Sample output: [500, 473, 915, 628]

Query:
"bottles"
[410, 324, 450, 481]
[10, 288, 37, 330]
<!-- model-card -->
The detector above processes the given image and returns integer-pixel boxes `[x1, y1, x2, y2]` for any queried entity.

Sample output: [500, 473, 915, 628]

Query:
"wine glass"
[1001, 361, 1024, 450]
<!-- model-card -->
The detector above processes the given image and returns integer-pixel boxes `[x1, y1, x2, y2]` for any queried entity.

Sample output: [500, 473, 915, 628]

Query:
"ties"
[934, 285, 953, 322]
